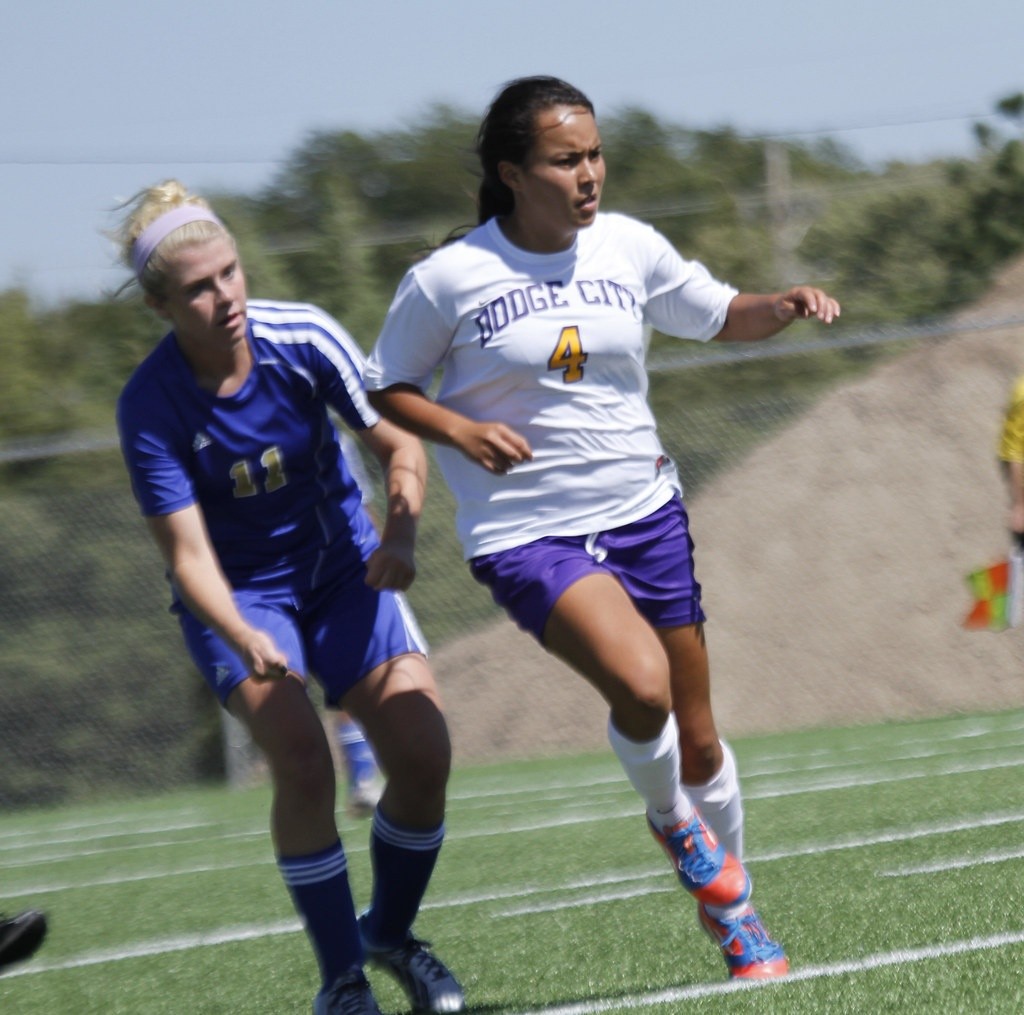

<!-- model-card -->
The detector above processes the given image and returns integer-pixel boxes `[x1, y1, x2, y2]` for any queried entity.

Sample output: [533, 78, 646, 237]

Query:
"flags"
[962, 558, 1021, 630]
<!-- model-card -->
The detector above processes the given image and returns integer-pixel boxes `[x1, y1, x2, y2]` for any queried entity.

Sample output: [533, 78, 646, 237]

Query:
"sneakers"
[315, 948, 380, 1015]
[647, 804, 752, 911]
[0, 911, 46, 967]
[698, 902, 789, 979]
[356, 911, 463, 1015]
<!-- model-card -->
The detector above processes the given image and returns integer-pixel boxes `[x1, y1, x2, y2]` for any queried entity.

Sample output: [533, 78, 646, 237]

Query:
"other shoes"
[345, 781, 385, 816]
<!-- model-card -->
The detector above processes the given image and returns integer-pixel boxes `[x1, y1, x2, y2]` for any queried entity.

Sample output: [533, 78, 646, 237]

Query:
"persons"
[999, 376, 1024, 551]
[0, 908, 48, 970]
[116, 184, 465, 1015]
[361, 77, 842, 982]
